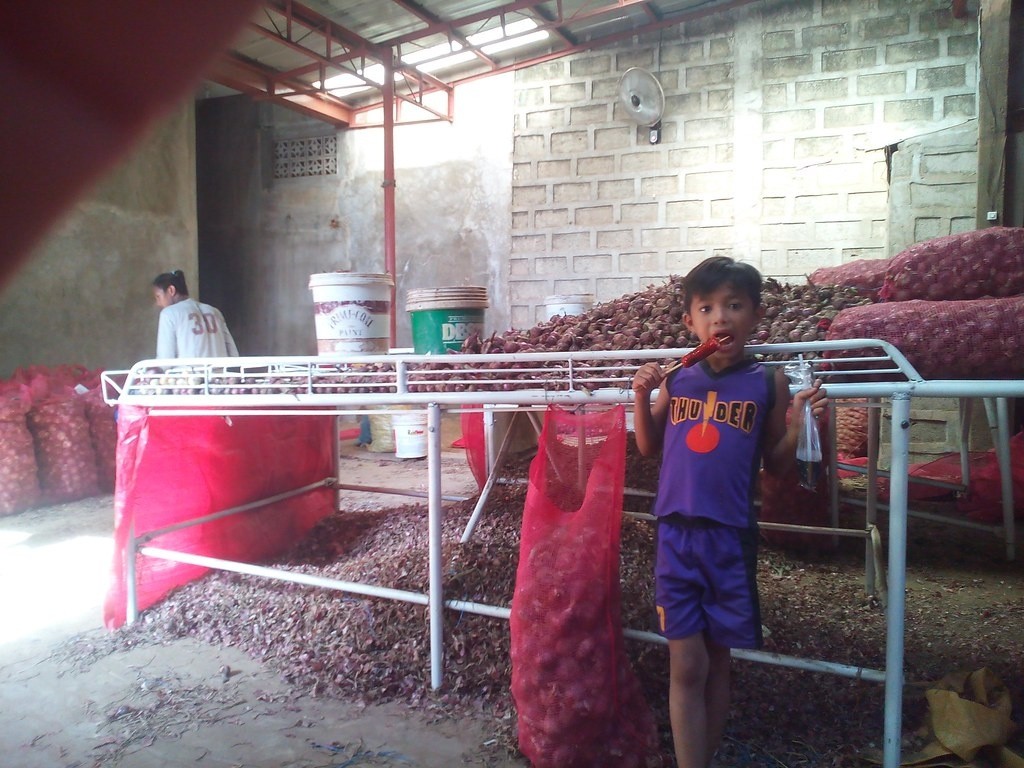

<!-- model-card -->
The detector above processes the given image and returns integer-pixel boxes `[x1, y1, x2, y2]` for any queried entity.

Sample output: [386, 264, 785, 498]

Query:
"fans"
[617, 65, 665, 146]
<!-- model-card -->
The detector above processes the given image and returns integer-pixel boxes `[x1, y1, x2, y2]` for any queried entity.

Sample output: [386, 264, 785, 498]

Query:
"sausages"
[682, 337, 720, 367]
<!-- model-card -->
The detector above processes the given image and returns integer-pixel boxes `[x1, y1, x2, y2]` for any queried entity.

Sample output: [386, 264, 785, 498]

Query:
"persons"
[630, 255, 831, 768]
[148, 267, 240, 360]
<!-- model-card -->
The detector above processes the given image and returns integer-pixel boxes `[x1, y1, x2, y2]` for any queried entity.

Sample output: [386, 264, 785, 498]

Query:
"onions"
[0, 379, 123, 517]
[509, 524, 659, 768]
[127, 226, 1024, 394]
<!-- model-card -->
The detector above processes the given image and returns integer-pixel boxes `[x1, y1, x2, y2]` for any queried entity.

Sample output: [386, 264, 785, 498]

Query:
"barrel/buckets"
[407, 286, 489, 355]
[392, 415, 428, 459]
[310, 274, 394, 356]
